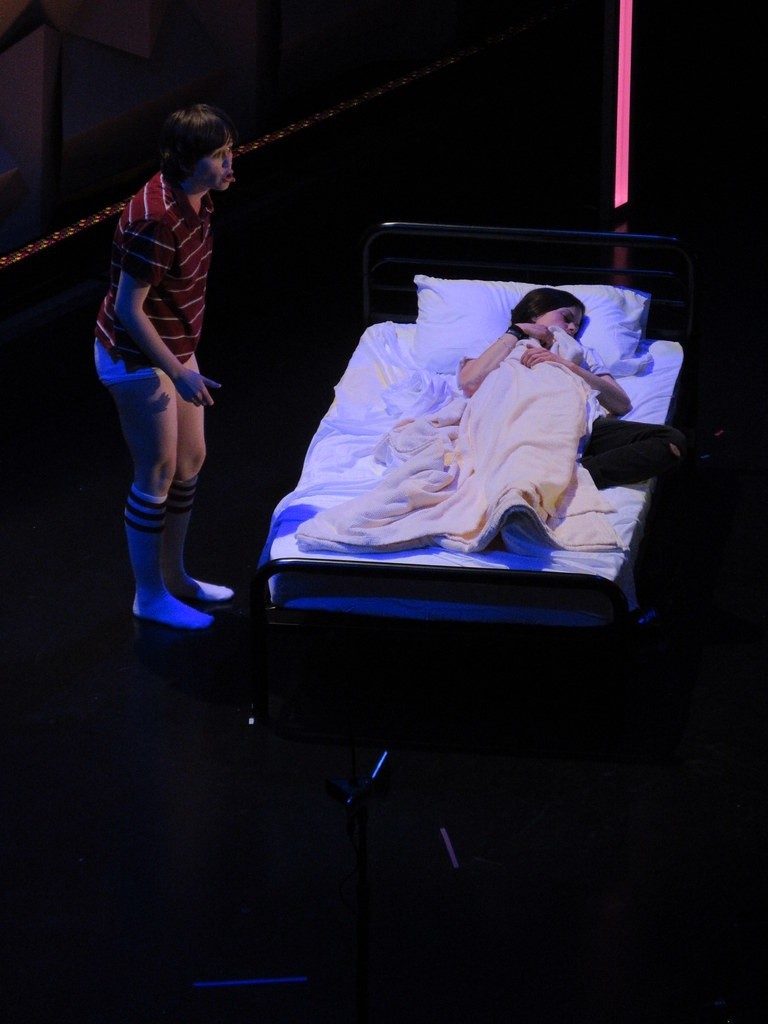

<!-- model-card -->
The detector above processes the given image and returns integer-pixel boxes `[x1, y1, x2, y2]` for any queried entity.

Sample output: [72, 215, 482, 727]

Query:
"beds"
[243, 215, 701, 758]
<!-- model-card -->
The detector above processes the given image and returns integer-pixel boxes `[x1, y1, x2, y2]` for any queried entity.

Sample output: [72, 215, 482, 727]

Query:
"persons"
[460, 287, 630, 415]
[95, 104, 236, 630]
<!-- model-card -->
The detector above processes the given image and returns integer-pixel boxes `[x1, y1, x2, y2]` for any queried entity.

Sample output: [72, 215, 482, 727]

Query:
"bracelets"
[499, 337, 514, 351]
[506, 324, 524, 340]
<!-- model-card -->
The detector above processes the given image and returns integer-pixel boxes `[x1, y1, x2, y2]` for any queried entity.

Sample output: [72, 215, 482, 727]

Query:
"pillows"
[412, 273, 653, 378]
